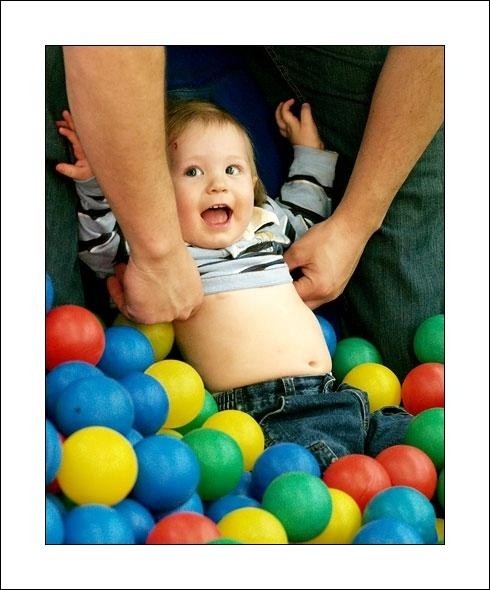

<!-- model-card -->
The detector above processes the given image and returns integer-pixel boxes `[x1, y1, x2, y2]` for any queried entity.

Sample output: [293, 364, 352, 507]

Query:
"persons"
[61, 46, 444, 325]
[55, 97, 415, 478]
